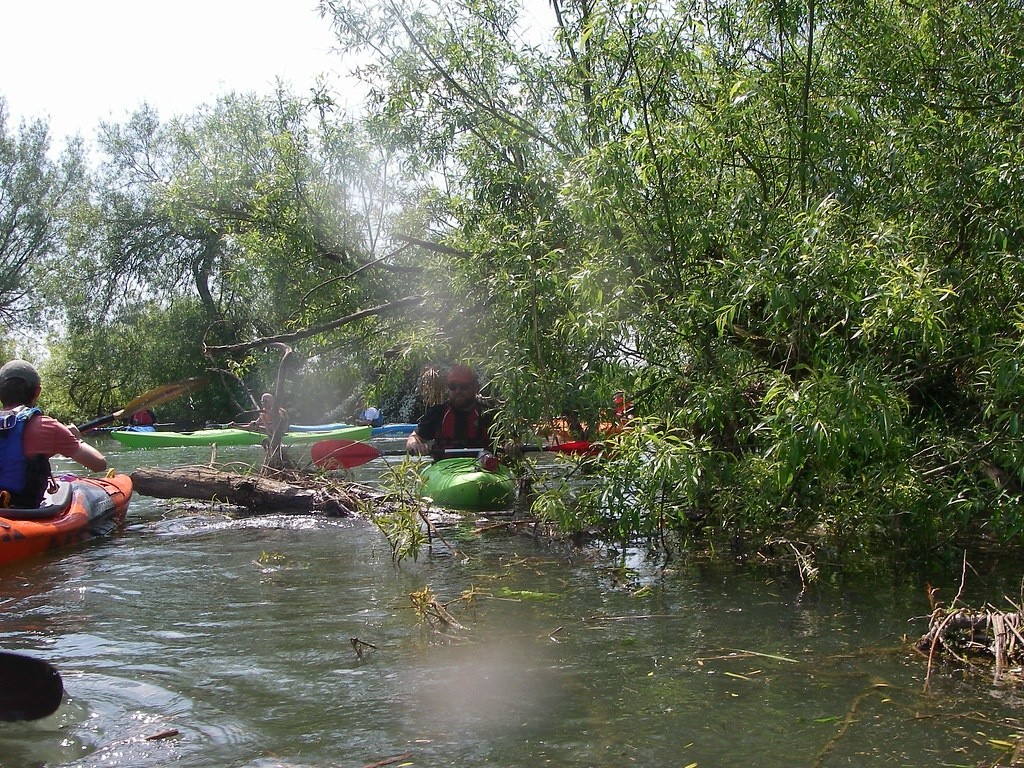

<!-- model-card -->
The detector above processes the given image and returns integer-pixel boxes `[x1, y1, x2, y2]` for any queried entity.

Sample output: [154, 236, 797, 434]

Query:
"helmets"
[0, 359, 41, 391]
[447, 363, 478, 387]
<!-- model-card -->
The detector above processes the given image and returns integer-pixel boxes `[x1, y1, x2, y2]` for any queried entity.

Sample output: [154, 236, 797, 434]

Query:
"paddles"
[311, 440, 617, 470]
[78, 375, 209, 434]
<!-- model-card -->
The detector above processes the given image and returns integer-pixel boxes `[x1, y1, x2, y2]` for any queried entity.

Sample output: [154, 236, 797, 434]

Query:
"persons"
[0, 360, 106, 508]
[355, 400, 383, 427]
[604, 392, 636, 439]
[231, 393, 282, 435]
[126, 407, 156, 426]
[406, 365, 524, 462]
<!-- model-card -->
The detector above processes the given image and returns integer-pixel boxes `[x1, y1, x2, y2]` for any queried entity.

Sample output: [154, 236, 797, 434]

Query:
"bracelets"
[81, 441, 85, 443]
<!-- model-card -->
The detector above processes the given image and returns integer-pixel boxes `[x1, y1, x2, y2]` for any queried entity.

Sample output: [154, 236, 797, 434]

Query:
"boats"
[290, 421, 420, 436]
[414, 458, 517, 512]
[538, 419, 622, 441]
[0, 466, 135, 566]
[110, 425, 373, 450]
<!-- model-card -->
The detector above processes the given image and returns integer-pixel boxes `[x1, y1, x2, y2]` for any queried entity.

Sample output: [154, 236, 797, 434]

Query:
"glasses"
[261, 398, 271, 402]
[447, 383, 473, 391]
